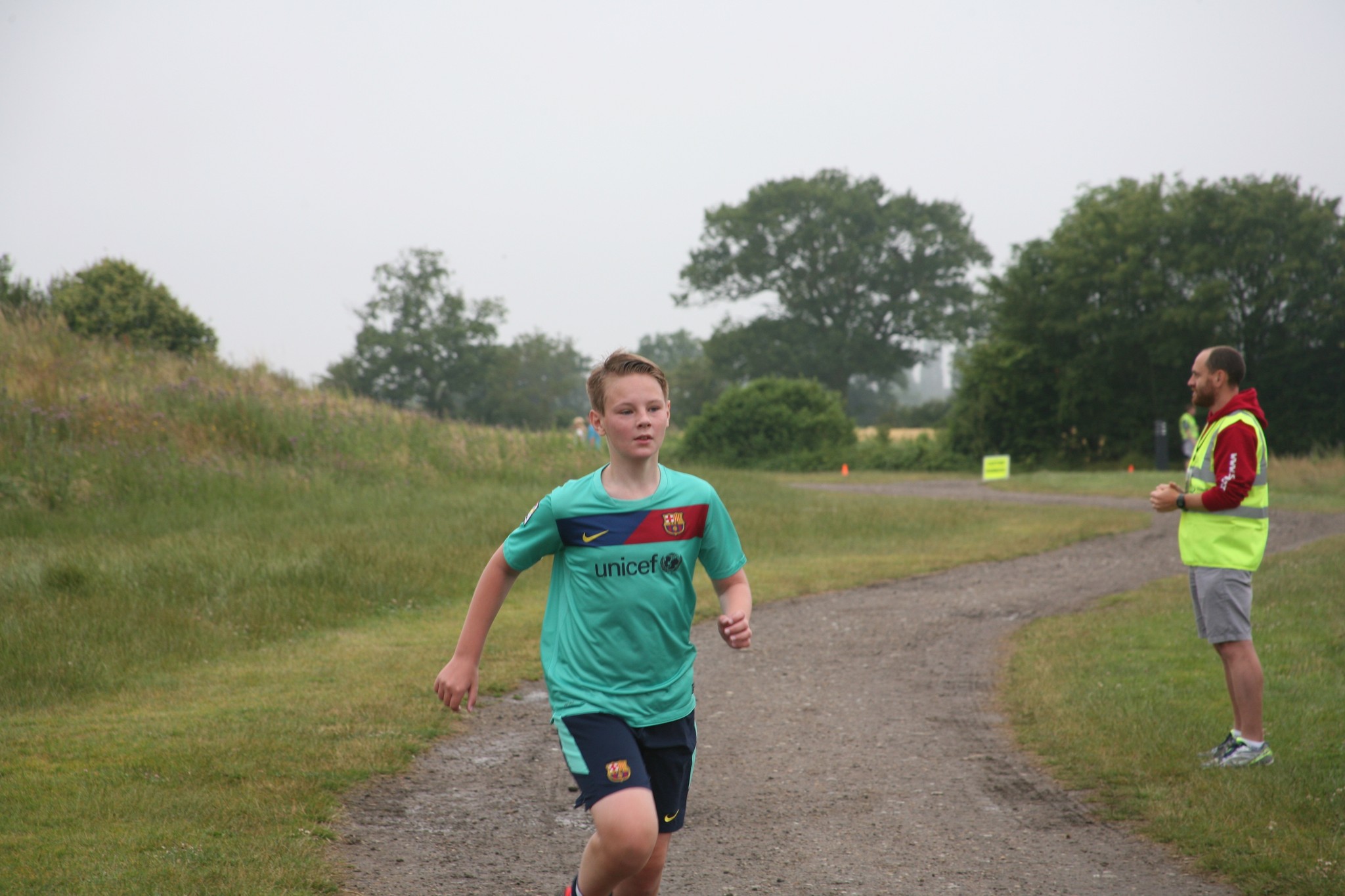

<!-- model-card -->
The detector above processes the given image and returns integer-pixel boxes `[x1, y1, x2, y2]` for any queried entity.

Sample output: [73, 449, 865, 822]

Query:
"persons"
[433, 347, 753, 896]
[1150, 345, 1275, 771]
[1179, 403, 1200, 470]
[572, 414, 600, 453]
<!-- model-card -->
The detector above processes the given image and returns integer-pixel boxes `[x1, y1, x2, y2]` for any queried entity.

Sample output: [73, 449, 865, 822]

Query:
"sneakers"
[1197, 729, 1238, 759]
[1201, 737, 1274, 767]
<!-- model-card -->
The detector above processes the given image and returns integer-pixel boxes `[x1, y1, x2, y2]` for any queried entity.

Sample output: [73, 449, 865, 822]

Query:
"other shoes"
[565, 875, 613, 896]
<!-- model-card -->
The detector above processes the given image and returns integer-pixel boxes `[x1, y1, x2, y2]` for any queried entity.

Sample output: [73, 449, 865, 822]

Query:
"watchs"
[1177, 492, 1188, 513]
